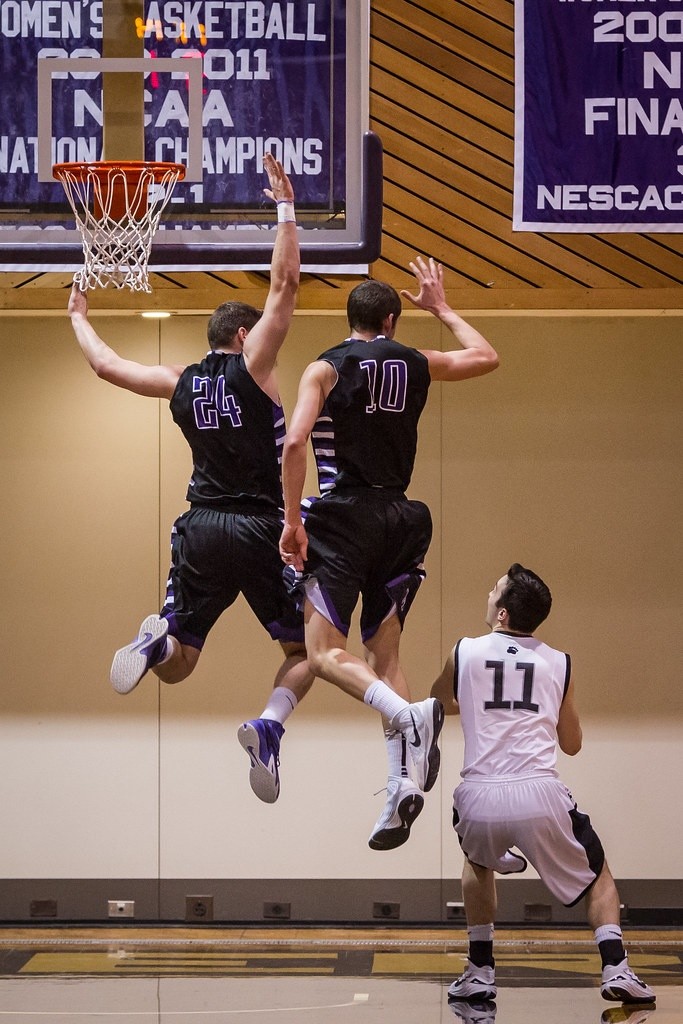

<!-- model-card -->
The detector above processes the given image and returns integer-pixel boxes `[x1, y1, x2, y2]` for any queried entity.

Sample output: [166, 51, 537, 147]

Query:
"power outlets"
[184, 895, 214, 923]
[263, 903, 290, 918]
[447, 902, 467, 919]
[374, 902, 400, 918]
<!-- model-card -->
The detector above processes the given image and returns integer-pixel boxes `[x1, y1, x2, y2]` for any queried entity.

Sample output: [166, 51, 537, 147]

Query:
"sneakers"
[369, 776, 425, 850]
[237, 718, 285, 804]
[446, 956, 498, 1001]
[448, 998, 497, 1024]
[110, 614, 170, 694]
[391, 696, 444, 793]
[601, 1003, 656, 1024]
[600, 949, 657, 1003]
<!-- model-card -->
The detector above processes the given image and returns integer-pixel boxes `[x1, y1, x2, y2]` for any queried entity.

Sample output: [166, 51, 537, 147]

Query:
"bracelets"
[276, 201, 297, 223]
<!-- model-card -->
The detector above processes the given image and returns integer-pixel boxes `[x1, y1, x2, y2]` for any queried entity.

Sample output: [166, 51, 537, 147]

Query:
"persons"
[68, 152, 315, 805]
[278, 255, 499, 850]
[427, 564, 659, 1004]
